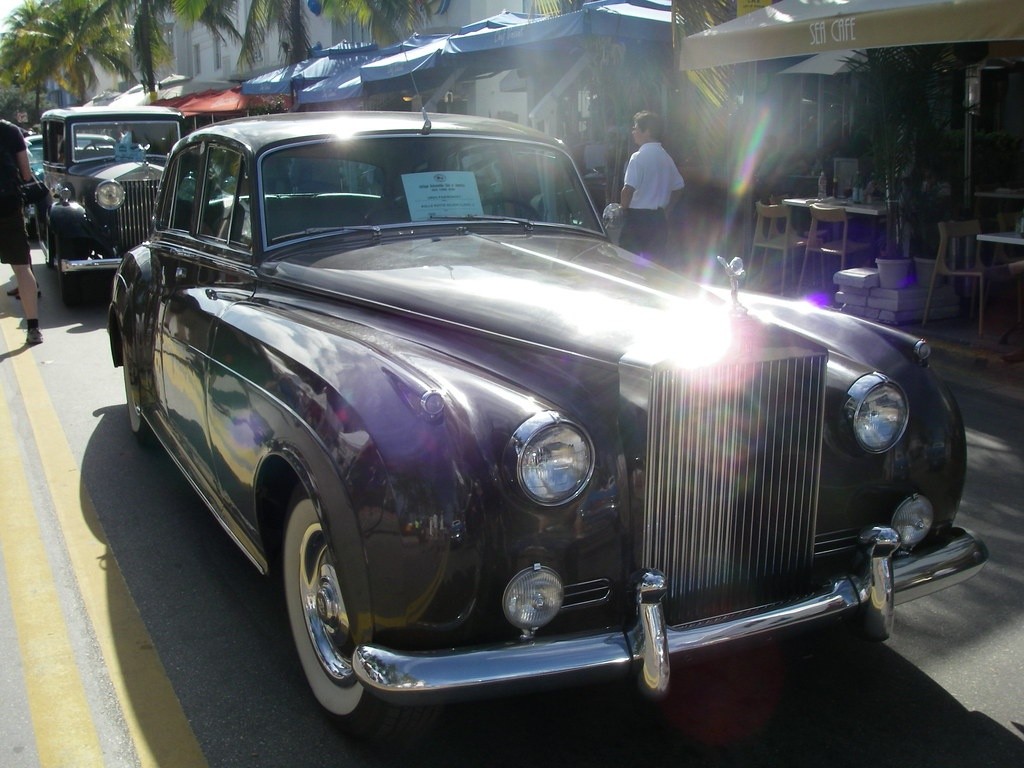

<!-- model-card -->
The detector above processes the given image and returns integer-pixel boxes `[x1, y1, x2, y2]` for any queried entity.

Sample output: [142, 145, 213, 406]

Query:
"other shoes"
[7, 282, 41, 299]
[26, 327, 43, 345]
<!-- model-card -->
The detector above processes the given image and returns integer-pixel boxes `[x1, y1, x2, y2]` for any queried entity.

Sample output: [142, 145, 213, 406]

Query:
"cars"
[33, 105, 228, 313]
[21, 133, 119, 244]
[106, 104, 991, 754]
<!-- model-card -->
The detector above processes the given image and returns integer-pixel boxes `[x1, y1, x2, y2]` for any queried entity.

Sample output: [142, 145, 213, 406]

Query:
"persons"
[620, 110, 686, 271]
[0, 119, 43, 346]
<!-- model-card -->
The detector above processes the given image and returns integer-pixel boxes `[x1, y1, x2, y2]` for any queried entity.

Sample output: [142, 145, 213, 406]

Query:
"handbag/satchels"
[18, 171, 49, 205]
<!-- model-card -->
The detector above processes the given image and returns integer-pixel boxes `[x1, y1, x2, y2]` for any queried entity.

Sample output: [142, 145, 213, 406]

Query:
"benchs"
[200, 195, 410, 235]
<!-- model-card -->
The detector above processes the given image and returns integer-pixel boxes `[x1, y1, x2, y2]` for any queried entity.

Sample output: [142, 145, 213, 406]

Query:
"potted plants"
[845, 45, 988, 289]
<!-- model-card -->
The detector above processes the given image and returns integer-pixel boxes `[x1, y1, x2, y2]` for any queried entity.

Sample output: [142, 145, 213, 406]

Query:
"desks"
[783, 197, 887, 215]
[975, 231, 1024, 245]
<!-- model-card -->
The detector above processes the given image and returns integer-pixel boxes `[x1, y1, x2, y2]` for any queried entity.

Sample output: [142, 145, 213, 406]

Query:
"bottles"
[852, 170, 863, 204]
[818, 171, 827, 199]
[1020, 210, 1024, 239]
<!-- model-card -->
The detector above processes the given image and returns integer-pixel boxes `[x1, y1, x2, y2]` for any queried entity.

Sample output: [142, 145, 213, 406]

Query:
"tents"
[87, 0, 676, 137]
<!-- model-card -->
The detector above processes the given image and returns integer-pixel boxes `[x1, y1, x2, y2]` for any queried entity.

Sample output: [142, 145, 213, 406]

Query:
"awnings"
[678, 0, 1024, 71]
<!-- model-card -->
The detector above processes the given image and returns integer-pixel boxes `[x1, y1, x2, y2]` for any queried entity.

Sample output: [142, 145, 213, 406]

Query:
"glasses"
[633, 127, 641, 132]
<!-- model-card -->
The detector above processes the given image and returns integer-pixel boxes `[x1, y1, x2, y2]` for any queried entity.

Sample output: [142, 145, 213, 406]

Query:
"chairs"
[795, 202, 874, 287]
[919, 219, 984, 339]
[982, 211, 1024, 323]
[752, 200, 825, 289]
